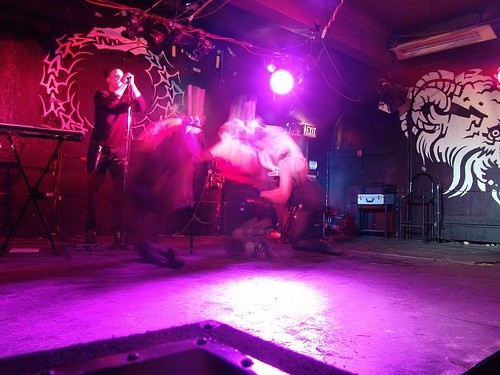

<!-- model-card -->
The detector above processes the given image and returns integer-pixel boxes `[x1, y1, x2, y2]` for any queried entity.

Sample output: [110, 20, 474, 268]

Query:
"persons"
[86, 66, 146, 249]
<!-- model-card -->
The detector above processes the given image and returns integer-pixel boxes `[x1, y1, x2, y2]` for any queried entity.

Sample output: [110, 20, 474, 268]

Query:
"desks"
[357, 204, 397, 238]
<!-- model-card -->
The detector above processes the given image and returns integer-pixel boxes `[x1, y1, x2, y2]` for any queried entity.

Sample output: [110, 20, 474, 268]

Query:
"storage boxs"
[358, 194, 394, 204]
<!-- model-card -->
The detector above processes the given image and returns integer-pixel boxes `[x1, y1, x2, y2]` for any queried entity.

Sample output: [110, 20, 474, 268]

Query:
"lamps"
[388, 23, 498, 60]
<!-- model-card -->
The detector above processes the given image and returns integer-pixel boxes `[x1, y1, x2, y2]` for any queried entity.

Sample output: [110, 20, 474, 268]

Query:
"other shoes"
[85, 227, 97, 244]
[113, 226, 127, 242]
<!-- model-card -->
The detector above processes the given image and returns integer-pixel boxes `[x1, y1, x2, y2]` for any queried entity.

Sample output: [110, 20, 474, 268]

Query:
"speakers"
[221, 182, 251, 236]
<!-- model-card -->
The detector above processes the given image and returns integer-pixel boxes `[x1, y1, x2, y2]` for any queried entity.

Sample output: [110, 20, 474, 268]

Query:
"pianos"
[0, 123, 85, 255]
[267, 171, 316, 237]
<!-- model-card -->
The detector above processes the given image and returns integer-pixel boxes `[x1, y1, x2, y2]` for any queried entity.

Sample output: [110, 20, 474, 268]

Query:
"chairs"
[323, 185, 361, 237]
[399, 173, 442, 243]
